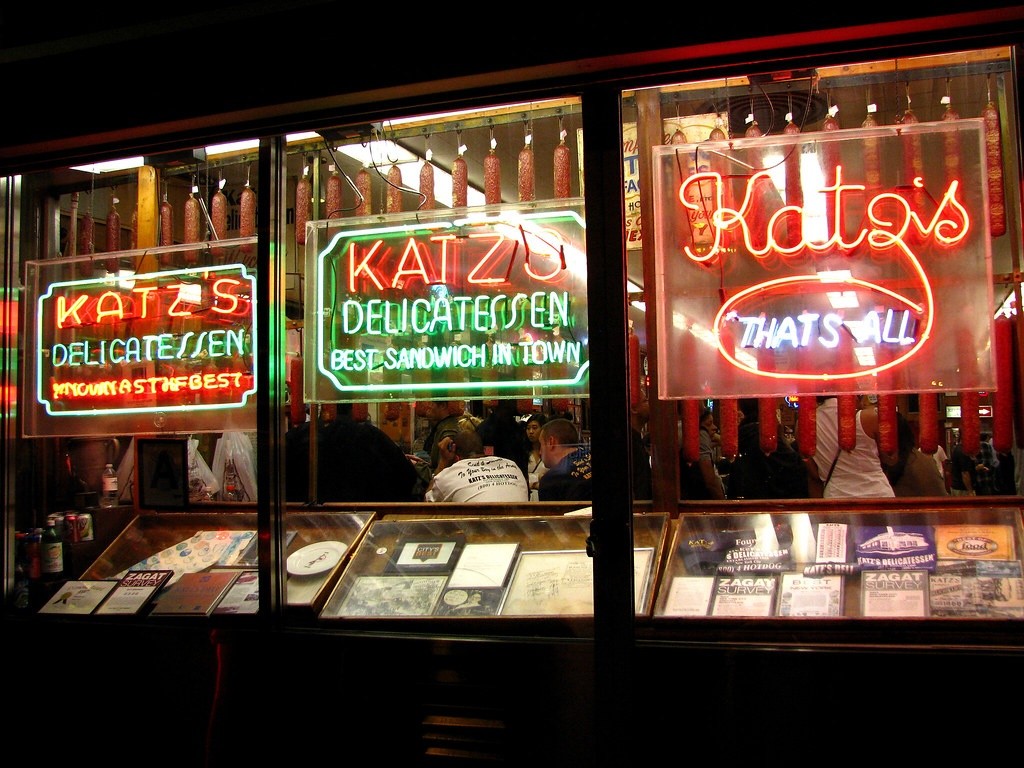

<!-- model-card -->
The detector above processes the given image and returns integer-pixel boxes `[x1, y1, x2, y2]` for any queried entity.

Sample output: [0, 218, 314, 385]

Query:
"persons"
[918, 445, 947, 478]
[527, 414, 550, 501]
[423, 430, 528, 502]
[716, 400, 798, 499]
[795, 395, 895, 498]
[405, 402, 482, 483]
[883, 412, 945, 495]
[538, 419, 591, 501]
[951, 431, 1016, 496]
[676, 400, 724, 500]
[446, 591, 482, 616]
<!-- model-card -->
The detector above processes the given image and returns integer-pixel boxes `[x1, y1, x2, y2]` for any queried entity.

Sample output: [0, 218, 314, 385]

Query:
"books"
[152, 571, 241, 614]
[663, 523, 1024, 616]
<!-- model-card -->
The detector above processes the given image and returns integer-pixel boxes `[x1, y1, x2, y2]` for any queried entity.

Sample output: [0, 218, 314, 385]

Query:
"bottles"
[42, 520, 65, 581]
[102, 464, 119, 509]
[15, 526, 44, 608]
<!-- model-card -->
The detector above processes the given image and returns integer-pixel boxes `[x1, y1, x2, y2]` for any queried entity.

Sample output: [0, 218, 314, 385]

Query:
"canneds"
[48, 513, 94, 546]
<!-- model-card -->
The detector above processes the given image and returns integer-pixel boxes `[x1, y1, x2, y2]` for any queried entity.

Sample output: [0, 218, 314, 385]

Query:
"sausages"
[671, 105, 1015, 464]
[292, 141, 640, 424]
[80, 188, 257, 275]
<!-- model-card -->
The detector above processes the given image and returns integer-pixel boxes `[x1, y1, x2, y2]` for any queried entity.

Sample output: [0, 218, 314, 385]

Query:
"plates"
[287, 540, 348, 575]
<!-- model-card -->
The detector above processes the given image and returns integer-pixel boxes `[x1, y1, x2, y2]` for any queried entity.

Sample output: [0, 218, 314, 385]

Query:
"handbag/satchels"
[210, 428, 258, 501]
[115, 442, 134, 502]
[183, 437, 219, 502]
